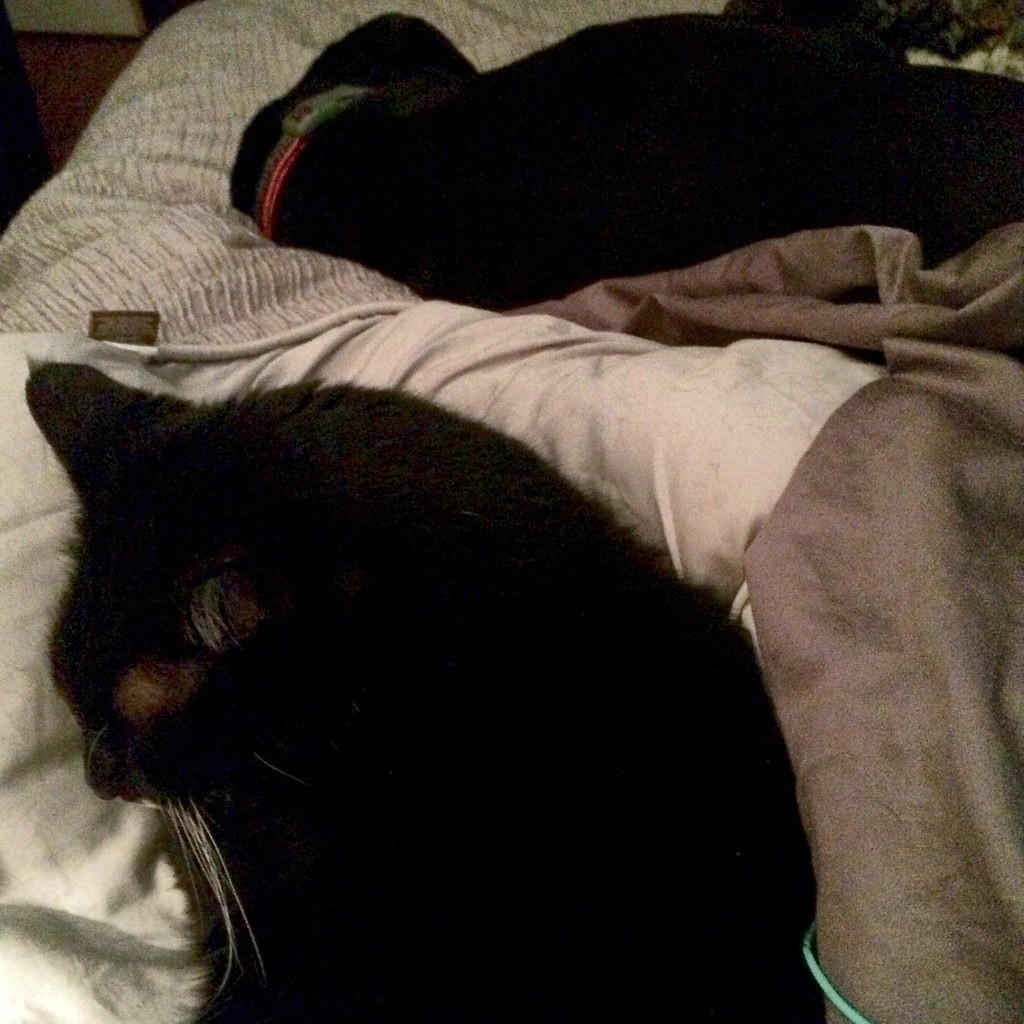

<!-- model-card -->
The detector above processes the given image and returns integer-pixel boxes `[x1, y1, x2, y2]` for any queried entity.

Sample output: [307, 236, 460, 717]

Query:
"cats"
[24, 352, 828, 1024]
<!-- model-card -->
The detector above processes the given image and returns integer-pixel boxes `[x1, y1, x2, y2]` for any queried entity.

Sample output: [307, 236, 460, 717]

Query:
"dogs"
[228, 10, 1024, 313]
